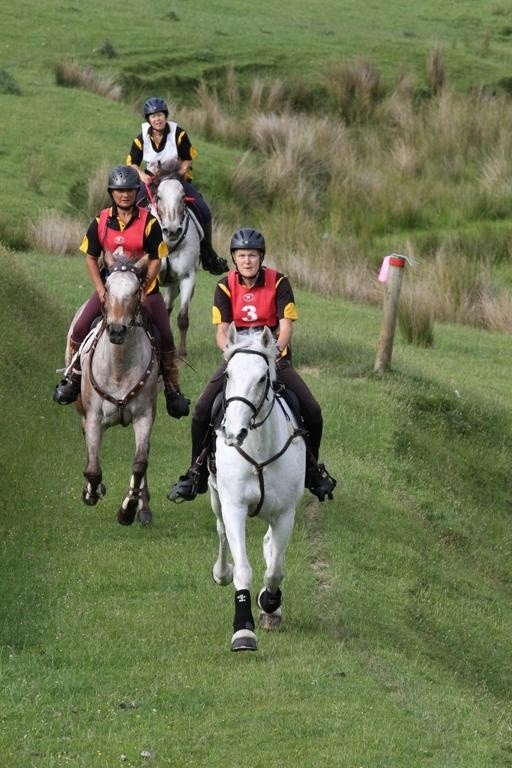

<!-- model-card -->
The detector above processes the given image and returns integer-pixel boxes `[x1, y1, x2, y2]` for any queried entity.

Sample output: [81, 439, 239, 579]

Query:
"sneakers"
[305, 467, 336, 489]
[179, 475, 208, 494]
[202, 255, 229, 272]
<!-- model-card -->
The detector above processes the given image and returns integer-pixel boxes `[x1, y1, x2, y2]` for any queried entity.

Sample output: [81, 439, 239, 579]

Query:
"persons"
[178, 228, 331, 501]
[126, 97, 230, 275]
[53, 165, 192, 418]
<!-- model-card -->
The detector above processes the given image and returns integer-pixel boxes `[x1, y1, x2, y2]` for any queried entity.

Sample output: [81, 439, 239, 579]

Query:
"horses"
[204, 318, 308, 654]
[63, 251, 165, 530]
[142, 157, 203, 369]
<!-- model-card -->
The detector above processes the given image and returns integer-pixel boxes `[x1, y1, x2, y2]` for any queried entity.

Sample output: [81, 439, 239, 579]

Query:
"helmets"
[230, 228, 265, 250]
[144, 98, 168, 115]
[107, 165, 140, 190]
